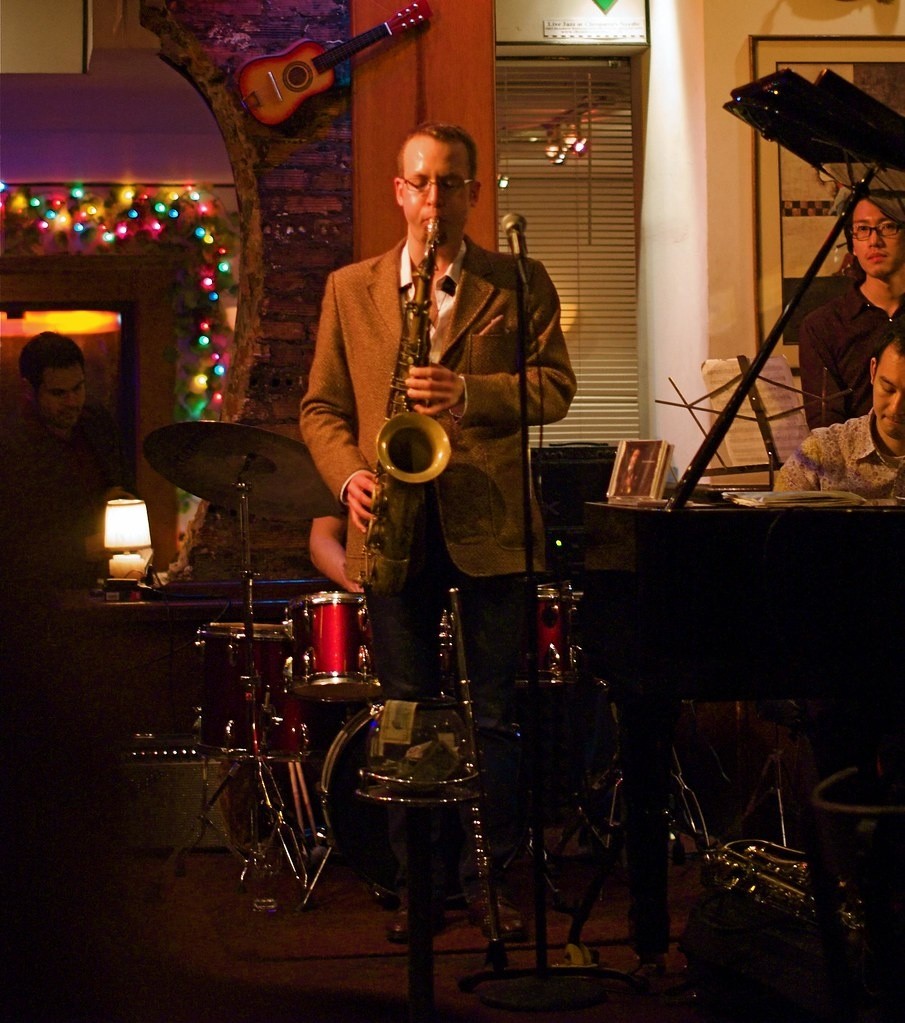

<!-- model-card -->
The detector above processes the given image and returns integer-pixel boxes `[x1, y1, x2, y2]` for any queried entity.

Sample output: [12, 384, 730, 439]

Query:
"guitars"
[232, 2, 432, 126]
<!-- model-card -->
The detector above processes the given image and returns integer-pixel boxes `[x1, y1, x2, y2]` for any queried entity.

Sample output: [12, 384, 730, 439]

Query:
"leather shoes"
[468, 880, 527, 939]
[388, 878, 443, 941]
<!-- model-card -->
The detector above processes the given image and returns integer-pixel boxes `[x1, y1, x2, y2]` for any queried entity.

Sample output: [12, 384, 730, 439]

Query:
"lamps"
[104, 499, 153, 583]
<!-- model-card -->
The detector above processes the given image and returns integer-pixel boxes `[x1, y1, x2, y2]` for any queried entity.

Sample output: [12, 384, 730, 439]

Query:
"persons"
[311, 516, 364, 593]
[1, 331, 133, 587]
[798, 198, 905, 433]
[774, 330, 905, 496]
[301, 123, 576, 942]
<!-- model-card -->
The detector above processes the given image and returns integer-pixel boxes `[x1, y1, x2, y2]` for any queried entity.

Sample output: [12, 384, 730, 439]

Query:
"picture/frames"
[747, 34, 904, 373]
[606, 437, 674, 509]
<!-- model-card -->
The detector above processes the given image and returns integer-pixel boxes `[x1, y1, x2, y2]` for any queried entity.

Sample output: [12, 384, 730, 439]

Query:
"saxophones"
[680, 839, 867, 937]
[367, 218, 451, 588]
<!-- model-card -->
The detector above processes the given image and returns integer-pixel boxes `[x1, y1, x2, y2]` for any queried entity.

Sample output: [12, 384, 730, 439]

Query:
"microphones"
[502, 212, 530, 283]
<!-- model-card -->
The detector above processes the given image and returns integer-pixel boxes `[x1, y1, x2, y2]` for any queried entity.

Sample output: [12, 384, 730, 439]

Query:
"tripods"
[175, 457, 316, 913]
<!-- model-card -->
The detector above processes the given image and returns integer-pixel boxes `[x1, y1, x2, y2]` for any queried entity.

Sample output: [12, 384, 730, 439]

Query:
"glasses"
[403, 176, 474, 196]
[849, 220, 904, 238]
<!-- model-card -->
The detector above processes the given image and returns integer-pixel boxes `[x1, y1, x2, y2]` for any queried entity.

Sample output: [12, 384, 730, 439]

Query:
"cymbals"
[141, 419, 349, 521]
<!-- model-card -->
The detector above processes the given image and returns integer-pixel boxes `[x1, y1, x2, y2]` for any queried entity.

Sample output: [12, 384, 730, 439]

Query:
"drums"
[281, 589, 382, 705]
[195, 622, 308, 760]
[303, 683, 481, 899]
[520, 589, 592, 688]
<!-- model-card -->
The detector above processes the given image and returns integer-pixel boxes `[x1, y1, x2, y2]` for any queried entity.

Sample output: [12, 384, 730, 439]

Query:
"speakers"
[113, 750, 248, 849]
[531, 446, 619, 573]
